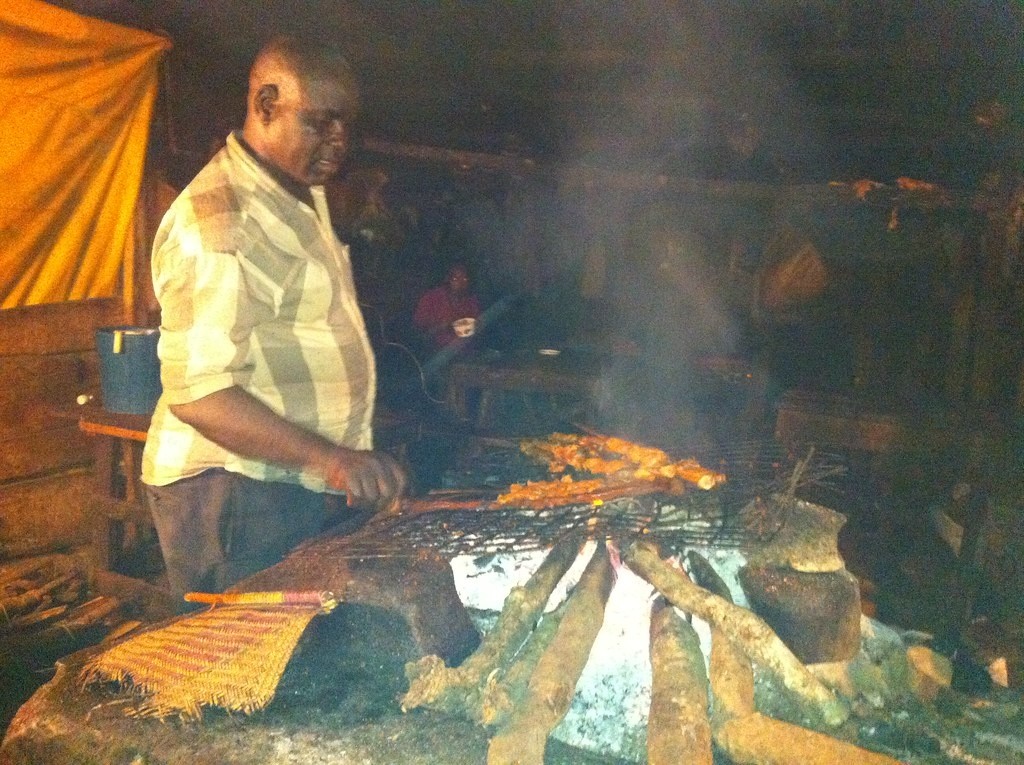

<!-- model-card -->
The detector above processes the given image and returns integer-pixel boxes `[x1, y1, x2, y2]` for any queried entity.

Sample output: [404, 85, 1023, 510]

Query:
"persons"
[409, 262, 485, 352]
[132, 35, 409, 606]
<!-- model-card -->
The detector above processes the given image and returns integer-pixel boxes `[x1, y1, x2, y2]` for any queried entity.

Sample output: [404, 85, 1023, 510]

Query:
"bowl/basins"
[454, 318, 475, 338]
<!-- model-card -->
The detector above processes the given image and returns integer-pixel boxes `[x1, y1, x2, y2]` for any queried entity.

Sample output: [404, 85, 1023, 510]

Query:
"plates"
[538, 347, 559, 355]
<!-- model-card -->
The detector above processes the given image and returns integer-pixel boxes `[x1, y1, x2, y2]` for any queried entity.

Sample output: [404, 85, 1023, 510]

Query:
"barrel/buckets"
[93, 324, 165, 416]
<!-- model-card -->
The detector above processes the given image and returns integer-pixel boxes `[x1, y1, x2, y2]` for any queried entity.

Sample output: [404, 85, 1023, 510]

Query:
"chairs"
[443, 360, 604, 435]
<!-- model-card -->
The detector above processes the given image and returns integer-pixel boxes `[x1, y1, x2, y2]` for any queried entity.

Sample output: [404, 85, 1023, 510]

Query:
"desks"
[76, 405, 169, 616]
